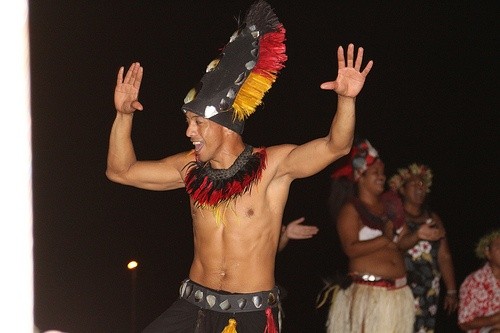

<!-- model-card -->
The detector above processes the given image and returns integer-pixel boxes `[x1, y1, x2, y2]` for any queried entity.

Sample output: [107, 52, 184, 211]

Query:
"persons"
[95, 0, 374, 332]
[390, 163, 461, 332]
[323, 138, 448, 332]
[457, 229, 500, 331]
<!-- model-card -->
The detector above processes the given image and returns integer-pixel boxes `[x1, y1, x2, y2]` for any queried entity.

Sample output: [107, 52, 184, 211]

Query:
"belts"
[178, 278, 285, 314]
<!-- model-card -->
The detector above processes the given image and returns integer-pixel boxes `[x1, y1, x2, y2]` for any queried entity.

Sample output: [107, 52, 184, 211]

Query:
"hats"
[182, 0, 289, 134]
